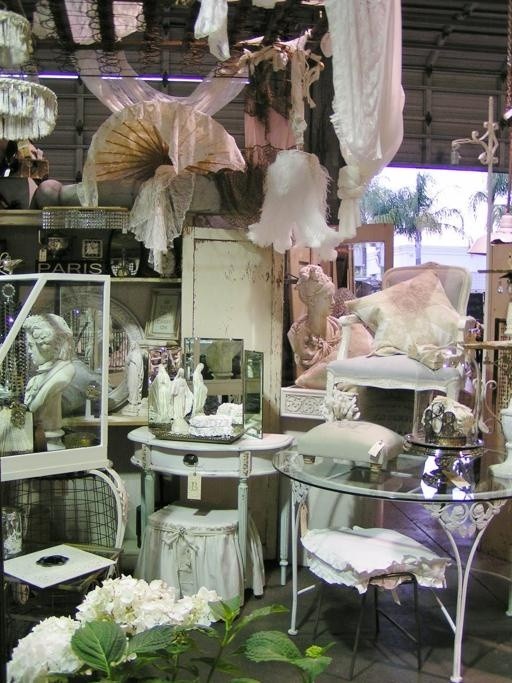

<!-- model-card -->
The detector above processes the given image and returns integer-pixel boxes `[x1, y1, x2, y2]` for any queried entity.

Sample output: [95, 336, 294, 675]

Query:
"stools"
[300, 527, 423, 682]
[133, 497, 267, 610]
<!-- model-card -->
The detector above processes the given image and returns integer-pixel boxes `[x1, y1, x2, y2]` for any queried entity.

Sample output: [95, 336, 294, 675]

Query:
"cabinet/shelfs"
[2, 209, 185, 427]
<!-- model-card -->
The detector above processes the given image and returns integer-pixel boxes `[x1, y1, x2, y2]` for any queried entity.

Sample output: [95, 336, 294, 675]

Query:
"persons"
[285, 264, 344, 365]
[21, 313, 91, 413]
[125, 340, 208, 436]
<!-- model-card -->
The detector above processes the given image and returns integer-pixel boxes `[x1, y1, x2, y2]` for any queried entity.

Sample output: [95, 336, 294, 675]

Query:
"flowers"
[6, 572, 339, 683]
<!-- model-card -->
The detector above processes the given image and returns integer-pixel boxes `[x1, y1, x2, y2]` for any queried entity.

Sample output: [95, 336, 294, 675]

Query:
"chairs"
[325, 263, 472, 432]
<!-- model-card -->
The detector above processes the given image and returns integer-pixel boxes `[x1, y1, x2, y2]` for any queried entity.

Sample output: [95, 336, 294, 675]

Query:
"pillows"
[343, 265, 468, 358]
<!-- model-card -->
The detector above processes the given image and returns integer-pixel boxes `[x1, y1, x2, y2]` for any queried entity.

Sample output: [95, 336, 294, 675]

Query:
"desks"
[124, 424, 298, 604]
[271, 445, 512, 683]
